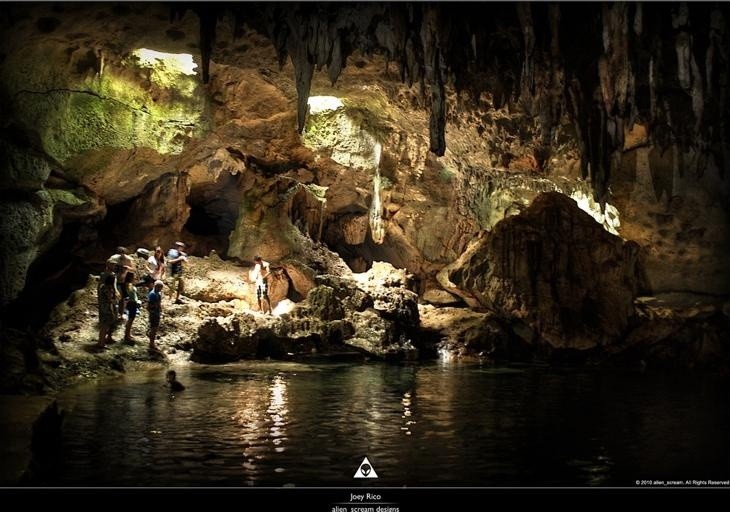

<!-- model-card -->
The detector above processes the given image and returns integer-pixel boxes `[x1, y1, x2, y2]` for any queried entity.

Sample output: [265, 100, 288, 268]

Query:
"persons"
[106, 259, 122, 343]
[106, 246, 134, 321]
[144, 246, 166, 282]
[167, 240, 188, 305]
[249, 253, 273, 316]
[124, 271, 138, 342]
[145, 280, 163, 350]
[94, 271, 117, 347]
[165, 369, 185, 395]
